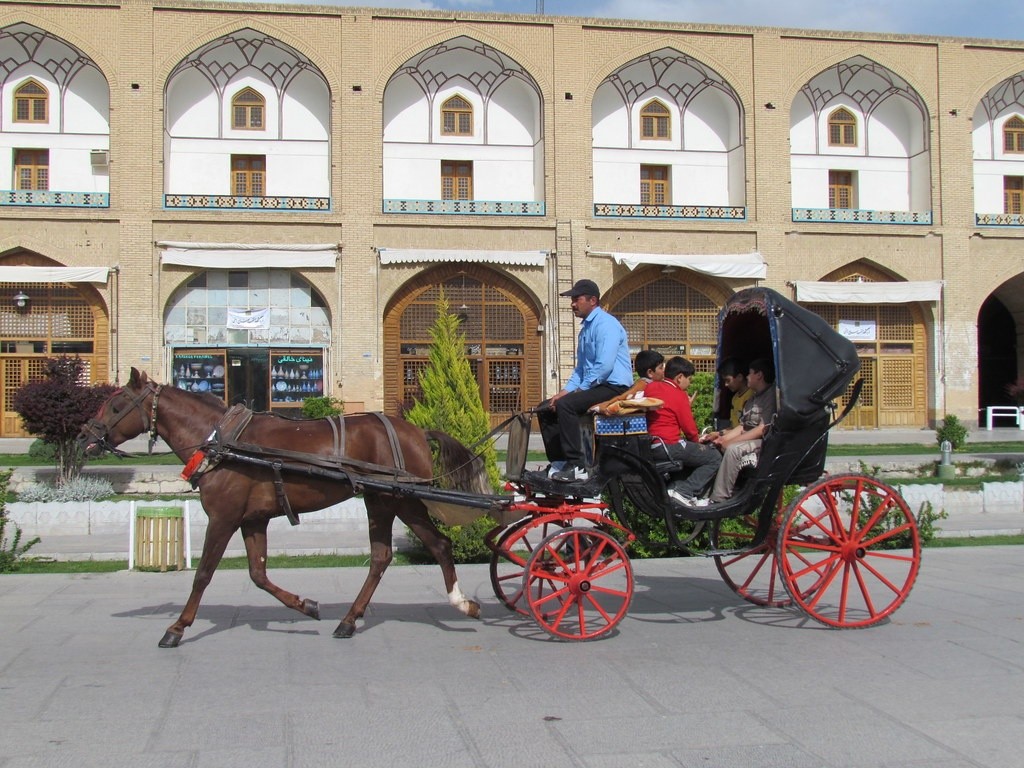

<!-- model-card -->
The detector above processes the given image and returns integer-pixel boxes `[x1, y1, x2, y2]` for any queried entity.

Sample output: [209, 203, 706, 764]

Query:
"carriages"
[75, 286, 924, 650]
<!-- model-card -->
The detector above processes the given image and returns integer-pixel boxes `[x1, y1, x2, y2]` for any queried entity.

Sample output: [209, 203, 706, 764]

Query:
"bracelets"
[718, 430, 723, 436]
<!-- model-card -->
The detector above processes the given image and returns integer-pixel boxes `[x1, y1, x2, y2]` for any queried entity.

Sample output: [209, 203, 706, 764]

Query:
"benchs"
[712, 413, 830, 488]
[619, 419, 683, 472]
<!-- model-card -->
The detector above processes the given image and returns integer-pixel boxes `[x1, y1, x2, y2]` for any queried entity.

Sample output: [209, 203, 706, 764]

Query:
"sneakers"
[552, 462, 589, 483]
[532, 463, 560, 479]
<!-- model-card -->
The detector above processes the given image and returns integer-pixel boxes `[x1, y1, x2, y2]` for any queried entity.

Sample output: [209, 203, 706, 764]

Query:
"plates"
[214, 365, 225, 378]
[199, 380, 208, 391]
[276, 381, 287, 391]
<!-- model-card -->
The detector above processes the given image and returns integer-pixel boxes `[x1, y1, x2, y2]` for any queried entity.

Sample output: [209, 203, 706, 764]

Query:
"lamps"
[458, 273, 470, 309]
[12, 290, 31, 307]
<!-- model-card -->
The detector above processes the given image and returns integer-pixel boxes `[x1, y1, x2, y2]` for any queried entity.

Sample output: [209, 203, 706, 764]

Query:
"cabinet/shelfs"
[271, 376, 323, 395]
[174, 376, 225, 396]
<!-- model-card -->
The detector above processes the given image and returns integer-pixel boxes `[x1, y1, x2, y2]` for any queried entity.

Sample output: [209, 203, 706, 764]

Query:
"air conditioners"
[90, 150, 109, 166]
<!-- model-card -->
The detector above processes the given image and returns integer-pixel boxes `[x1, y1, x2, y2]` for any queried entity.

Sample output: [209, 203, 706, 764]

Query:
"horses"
[77, 366, 497, 649]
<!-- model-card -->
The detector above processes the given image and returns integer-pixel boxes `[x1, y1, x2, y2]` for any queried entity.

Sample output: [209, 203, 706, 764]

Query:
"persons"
[632, 350, 723, 509]
[695, 360, 776, 506]
[536, 279, 634, 482]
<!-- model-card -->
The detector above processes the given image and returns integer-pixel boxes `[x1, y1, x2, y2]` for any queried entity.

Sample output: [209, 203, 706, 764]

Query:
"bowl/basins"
[212, 383, 224, 388]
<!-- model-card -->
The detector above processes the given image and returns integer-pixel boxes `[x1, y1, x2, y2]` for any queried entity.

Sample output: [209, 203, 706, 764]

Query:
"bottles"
[191, 380, 199, 392]
[186, 362, 191, 377]
[271, 366, 323, 402]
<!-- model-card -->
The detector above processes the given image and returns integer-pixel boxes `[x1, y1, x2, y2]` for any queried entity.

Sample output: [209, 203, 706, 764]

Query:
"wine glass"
[191, 363, 203, 378]
[204, 365, 214, 378]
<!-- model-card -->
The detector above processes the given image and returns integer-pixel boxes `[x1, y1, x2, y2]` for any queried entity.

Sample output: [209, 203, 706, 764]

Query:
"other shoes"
[690, 496, 716, 508]
[667, 488, 696, 510]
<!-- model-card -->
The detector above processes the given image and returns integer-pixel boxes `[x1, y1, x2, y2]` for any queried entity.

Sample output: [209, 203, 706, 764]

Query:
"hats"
[560, 279, 600, 299]
